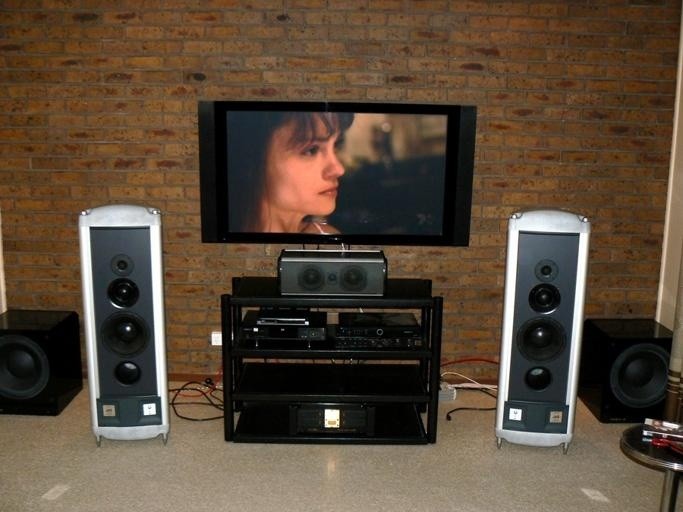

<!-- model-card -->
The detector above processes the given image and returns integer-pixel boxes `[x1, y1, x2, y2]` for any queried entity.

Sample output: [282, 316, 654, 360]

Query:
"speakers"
[495, 208, 591, 455]
[0, 308, 84, 417]
[578, 316, 674, 424]
[77, 202, 171, 447]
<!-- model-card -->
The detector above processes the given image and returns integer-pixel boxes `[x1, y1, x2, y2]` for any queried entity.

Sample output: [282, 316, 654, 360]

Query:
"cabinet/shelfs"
[221, 276, 444, 446]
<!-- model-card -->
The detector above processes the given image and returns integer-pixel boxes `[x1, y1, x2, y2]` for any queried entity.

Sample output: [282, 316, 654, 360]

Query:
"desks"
[621, 424, 683, 511]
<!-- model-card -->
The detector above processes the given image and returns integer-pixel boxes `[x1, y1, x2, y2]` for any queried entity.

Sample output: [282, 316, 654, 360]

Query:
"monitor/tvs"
[198, 99, 477, 247]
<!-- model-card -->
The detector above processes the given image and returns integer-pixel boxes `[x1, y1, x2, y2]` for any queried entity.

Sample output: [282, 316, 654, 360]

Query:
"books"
[643, 417, 683, 440]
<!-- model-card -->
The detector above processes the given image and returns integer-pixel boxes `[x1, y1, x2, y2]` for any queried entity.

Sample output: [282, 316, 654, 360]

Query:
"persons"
[225, 111, 354, 233]
